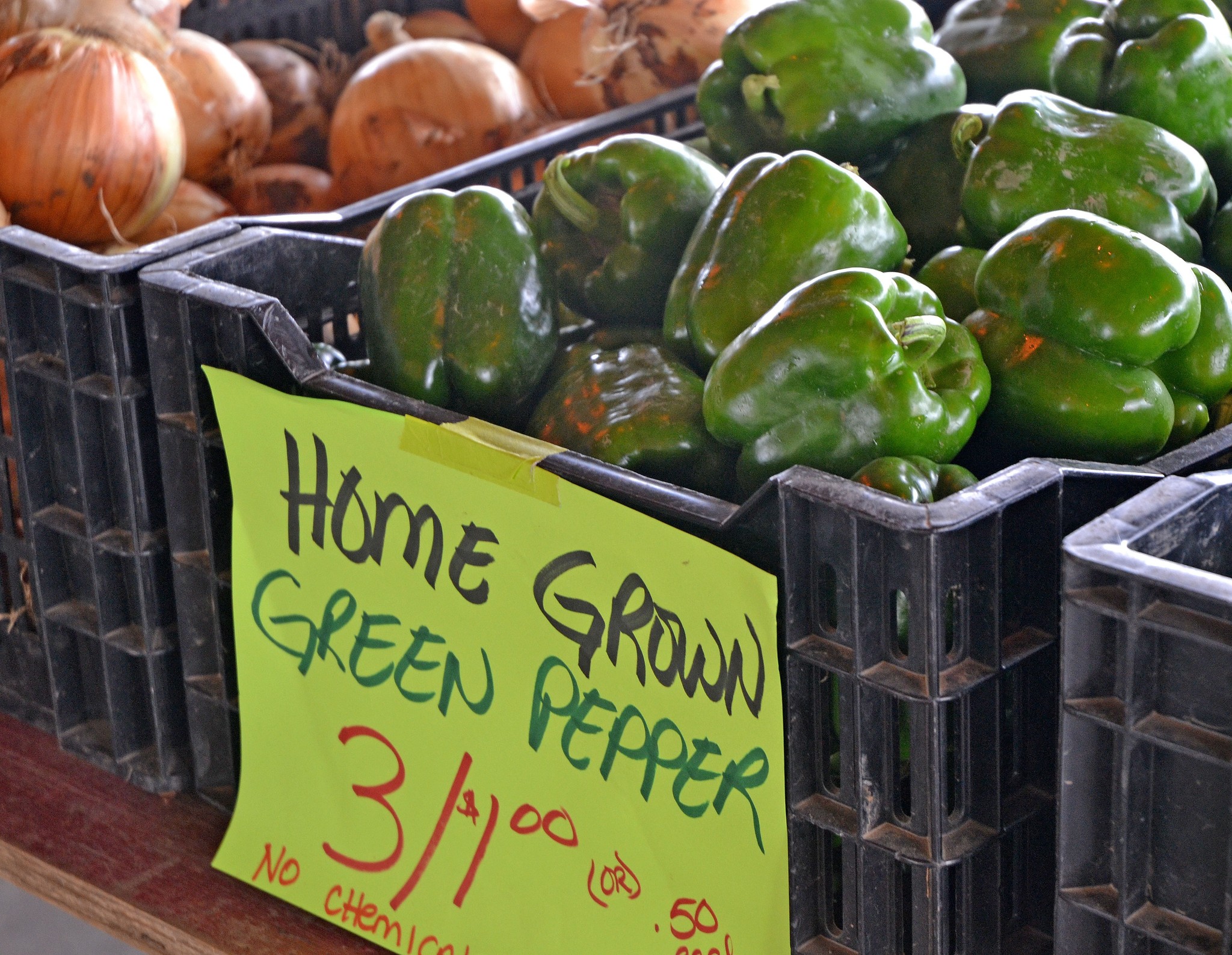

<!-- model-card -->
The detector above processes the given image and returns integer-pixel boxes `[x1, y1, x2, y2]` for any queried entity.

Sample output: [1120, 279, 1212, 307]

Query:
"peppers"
[356, 0, 1232, 766]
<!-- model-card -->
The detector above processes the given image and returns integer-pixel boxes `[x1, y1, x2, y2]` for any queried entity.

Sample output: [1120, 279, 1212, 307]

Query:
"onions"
[0, 0, 796, 535]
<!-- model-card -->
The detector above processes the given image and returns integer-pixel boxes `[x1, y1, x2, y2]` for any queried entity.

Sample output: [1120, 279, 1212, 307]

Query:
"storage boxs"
[0, 1, 1232, 955]
